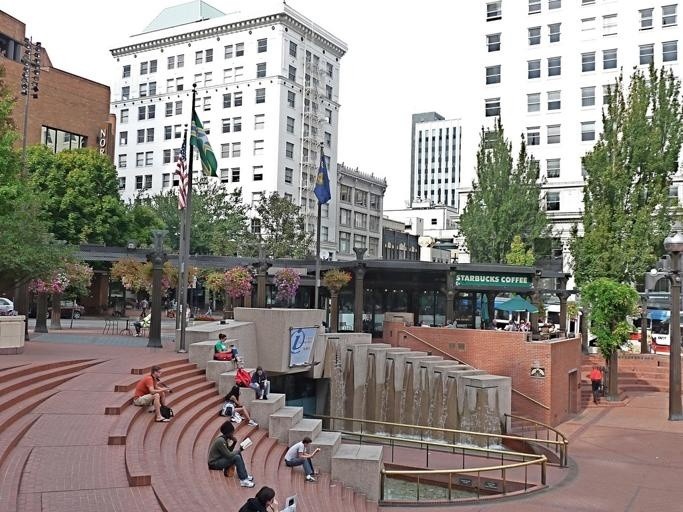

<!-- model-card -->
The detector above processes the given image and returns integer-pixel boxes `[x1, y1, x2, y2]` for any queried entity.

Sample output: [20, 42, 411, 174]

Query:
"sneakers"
[248, 422, 257, 426]
[305, 477, 315, 482]
[240, 475, 255, 488]
[156, 418, 170, 422]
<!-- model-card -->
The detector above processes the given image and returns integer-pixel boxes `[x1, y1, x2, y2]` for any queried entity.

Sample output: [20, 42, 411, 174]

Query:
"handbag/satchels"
[214, 352, 233, 360]
[160, 406, 170, 419]
[225, 465, 235, 476]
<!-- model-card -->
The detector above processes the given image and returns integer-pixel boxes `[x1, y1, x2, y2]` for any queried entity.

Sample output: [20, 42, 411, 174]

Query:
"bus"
[456, 298, 682, 357]
[320, 291, 456, 332]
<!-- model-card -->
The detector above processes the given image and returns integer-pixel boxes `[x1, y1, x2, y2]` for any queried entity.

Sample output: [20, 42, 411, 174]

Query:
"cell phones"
[229, 345, 234, 347]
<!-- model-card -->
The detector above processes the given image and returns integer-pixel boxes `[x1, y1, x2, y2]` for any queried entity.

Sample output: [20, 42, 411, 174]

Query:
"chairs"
[100, 309, 149, 338]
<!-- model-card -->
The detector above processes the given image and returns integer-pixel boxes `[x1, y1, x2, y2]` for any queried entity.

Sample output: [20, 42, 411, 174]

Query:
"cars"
[44, 300, 85, 320]
[0, 297, 13, 315]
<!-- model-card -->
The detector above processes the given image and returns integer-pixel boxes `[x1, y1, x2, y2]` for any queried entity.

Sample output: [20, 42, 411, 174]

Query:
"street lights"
[18, 31, 45, 190]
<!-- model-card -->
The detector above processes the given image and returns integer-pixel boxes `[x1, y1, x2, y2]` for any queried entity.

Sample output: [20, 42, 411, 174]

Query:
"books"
[239, 437, 254, 451]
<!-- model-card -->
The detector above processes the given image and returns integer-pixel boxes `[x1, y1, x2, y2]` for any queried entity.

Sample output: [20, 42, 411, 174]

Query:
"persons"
[133, 296, 191, 336]
[131, 365, 175, 423]
[420, 301, 557, 340]
[205, 298, 212, 317]
[221, 386, 258, 426]
[237, 486, 280, 512]
[249, 365, 272, 401]
[214, 333, 245, 370]
[207, 420, 256, 488]
[284, 436, 321, 483]
[591, 365, 606, 405]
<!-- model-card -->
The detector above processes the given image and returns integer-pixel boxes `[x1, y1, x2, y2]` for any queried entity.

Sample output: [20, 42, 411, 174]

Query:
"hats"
[221, 421, 234, 433]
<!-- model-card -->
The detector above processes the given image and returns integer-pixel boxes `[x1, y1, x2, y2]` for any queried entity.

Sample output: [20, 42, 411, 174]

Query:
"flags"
[313, 152, 331, 205]
[189, 107, 218, 178]
[173, 131, 191, 210]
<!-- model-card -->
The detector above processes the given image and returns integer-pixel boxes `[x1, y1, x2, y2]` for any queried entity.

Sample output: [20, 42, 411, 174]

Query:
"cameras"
[167, 390, 172, 394]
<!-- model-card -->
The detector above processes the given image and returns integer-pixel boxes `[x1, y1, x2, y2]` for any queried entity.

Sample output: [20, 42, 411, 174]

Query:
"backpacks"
[221, 401, 234, 416]
[236, 367, 251, 387]
